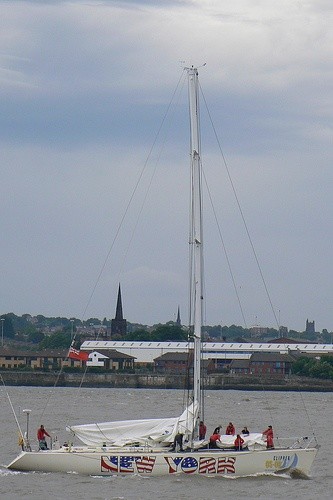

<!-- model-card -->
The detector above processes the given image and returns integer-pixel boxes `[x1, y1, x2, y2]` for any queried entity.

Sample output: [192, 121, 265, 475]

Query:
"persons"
[225, 422, 235, 435]
[37, 425, 51, 450]
[242, 427, 249, 434]
[173, 434, 184, 452]
[199, 421, 206, 440]
[263, 425, 275, 450]
[210, 425, 222, 449]
[235, 435, 244, 451]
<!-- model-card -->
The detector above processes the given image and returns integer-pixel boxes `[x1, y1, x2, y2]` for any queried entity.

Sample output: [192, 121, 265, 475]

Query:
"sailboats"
[3, 65, 322, 481]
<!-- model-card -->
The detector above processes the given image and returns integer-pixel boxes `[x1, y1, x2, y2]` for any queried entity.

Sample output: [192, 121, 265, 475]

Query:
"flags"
[67, 347, 90, 360]
